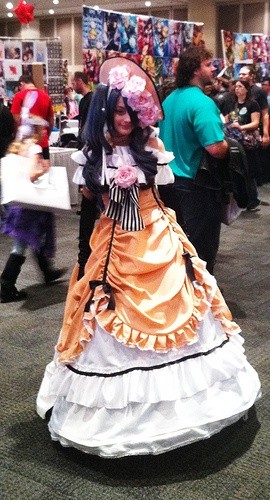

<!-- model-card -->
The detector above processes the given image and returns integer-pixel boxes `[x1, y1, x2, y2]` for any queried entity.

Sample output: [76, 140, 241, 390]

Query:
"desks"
[49, 148, 80, 206]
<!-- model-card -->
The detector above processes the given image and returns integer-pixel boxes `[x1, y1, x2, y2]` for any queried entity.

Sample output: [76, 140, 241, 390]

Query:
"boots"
[33, 246, 67, 282]
[0, 253, 26, 302]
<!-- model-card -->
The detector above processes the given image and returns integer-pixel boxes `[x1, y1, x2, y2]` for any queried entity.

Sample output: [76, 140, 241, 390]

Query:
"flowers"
[115, 163, 138, 189]
[108, 65, 160, 127]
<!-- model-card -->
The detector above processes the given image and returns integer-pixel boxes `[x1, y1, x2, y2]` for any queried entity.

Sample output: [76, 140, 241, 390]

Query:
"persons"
[35, 58, 263, 462]
[0, 45, 270, 304]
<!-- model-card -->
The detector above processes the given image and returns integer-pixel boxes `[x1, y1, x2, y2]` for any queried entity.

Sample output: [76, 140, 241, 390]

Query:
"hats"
[98, 57, 165, 130]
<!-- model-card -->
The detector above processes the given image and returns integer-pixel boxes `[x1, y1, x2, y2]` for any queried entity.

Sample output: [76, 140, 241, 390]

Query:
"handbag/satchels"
[0, 154, 71, 211]
[240, 127, 262, 149]
